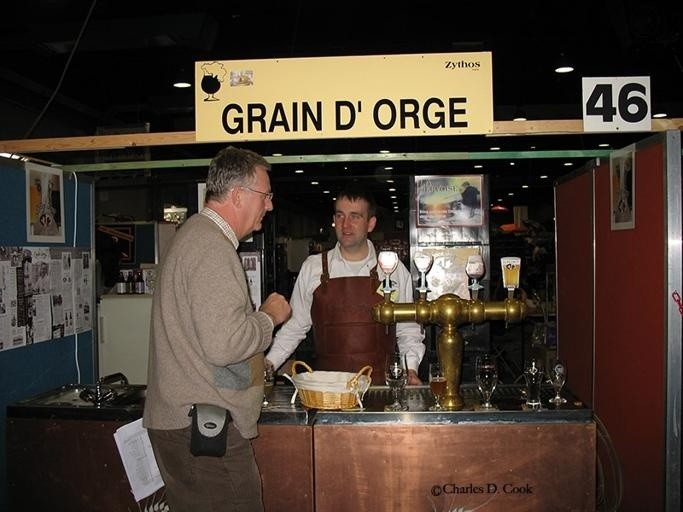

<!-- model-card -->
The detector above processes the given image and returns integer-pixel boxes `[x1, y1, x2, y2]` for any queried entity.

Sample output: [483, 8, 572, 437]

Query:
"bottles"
[117, 270, 146, 294]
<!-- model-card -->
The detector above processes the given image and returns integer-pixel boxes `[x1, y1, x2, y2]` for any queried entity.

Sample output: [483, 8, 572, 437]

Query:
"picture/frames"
[24, 161, 66, 245]
[609, 142, 637, 231]
[413, 173, 485, 229]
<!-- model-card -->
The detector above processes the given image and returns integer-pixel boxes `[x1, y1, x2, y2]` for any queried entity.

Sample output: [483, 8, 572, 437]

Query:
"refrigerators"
[95, 292, 154, 386]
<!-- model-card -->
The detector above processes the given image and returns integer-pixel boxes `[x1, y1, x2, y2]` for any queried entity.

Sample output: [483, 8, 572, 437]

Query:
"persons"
[458, 181, 479, 219]
[262, 185, 426, 384]
[141, 146, 292, 511]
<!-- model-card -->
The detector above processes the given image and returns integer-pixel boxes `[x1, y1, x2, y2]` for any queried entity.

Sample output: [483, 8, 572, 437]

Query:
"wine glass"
[378, 250, 397, 293]
[466, 254, 487, 290]
[413, 253, 435, 291]
[383, 352, 410, 414]
[428, 362, 449, 413]
[474, 355, 499, 410]
[548, 358, 568, 404]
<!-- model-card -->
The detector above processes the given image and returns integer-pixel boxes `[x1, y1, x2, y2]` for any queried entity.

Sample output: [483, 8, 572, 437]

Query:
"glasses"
[230, 186, 273, 202]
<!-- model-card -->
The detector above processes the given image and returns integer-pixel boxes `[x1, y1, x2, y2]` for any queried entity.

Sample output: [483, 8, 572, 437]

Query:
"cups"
[523, 357, 545, 406]
[264, 358, 277, 406]
[500, 255, 522, 291]
